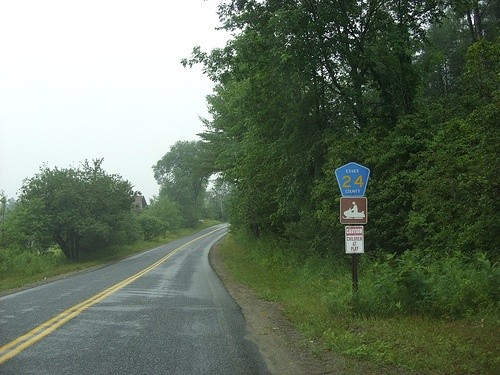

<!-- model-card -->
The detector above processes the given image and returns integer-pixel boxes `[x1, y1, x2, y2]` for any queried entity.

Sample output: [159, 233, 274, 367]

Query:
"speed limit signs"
[334, 162, 370, 197]
